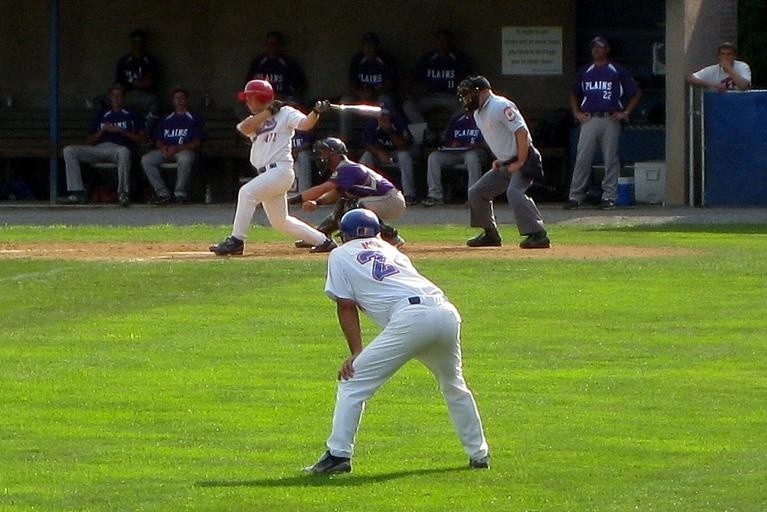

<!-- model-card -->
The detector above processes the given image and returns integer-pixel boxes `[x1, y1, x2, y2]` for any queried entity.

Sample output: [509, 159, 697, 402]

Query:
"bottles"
[203, 91, 210, 112]
[204, 185, 211, 204]
[5, 92, 12, 107]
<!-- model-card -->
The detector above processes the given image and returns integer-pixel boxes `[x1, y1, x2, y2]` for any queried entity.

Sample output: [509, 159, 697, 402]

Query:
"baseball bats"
[316, 101, 382, 116]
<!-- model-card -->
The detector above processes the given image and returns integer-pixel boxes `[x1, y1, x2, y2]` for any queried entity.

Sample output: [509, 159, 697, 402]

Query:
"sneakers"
[383, 233, 406, 250]
[470, 456, 490, 470]
[209, 236, 245, 256]
[465, 232, 502, 247]
[148, 193, 191, 204]
[302, 451, 352, 478]
[403, 195, 444, 207]
[56, 192, 90, 204]
[294, 240, 337, 253]
[599, 198, 615, 211]
[519, 235, 551, 248]
[118, 192, 130, 207]
[564, 198, 582, 210]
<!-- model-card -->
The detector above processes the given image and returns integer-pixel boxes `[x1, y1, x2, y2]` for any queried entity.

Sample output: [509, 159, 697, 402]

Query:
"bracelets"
[623, 111, 630, 115]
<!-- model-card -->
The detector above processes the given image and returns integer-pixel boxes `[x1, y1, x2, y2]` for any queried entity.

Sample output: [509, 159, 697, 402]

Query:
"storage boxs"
[614, 176, 635, 206]
[633, 162, 666, 205]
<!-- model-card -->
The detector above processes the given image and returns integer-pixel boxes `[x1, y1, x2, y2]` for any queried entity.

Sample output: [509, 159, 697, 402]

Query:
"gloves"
[267, 100, 283, 116]
[313, 100, 332, 115]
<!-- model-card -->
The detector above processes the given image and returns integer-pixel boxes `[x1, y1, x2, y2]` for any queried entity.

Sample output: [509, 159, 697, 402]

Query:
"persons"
[140, 85, 201, 205]
[210, 79, 337, 256]
[287, 136, 406, 249]
[457, 75, 549, 248]
[564, 36, 643, 211]
[338, 27, 467, 146]
[286, 82, 490, 205]
[240, 30, 310, 149]
[113, 30, 162, 146]
[55, 83, 145, 206]
[309, 207, 491, 477]
[687, 41, 752, 93]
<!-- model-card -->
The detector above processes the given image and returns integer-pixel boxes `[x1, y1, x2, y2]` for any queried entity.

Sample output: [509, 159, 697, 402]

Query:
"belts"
[585, 111, 614, 117]
[256, 162, 277, 174]
[500, 145, 535, 166]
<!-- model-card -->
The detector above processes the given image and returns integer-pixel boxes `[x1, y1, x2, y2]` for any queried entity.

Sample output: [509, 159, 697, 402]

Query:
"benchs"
[0, 111, 573, 206]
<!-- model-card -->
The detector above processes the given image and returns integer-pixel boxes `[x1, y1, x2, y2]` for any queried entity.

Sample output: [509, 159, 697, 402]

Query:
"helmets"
[237, 78, 274, 100]
[338, 207, 382, 239]
[455, 75, 492, 113]
[311, 136, 350, 177]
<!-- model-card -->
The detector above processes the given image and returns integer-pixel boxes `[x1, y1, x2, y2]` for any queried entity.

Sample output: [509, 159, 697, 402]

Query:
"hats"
[108, 81, 127, 95]
[170, 84, 189, 98]
[589, 36, 610, 48]
[376, 97, 394, 115]
[358, 31, 380, 46]
